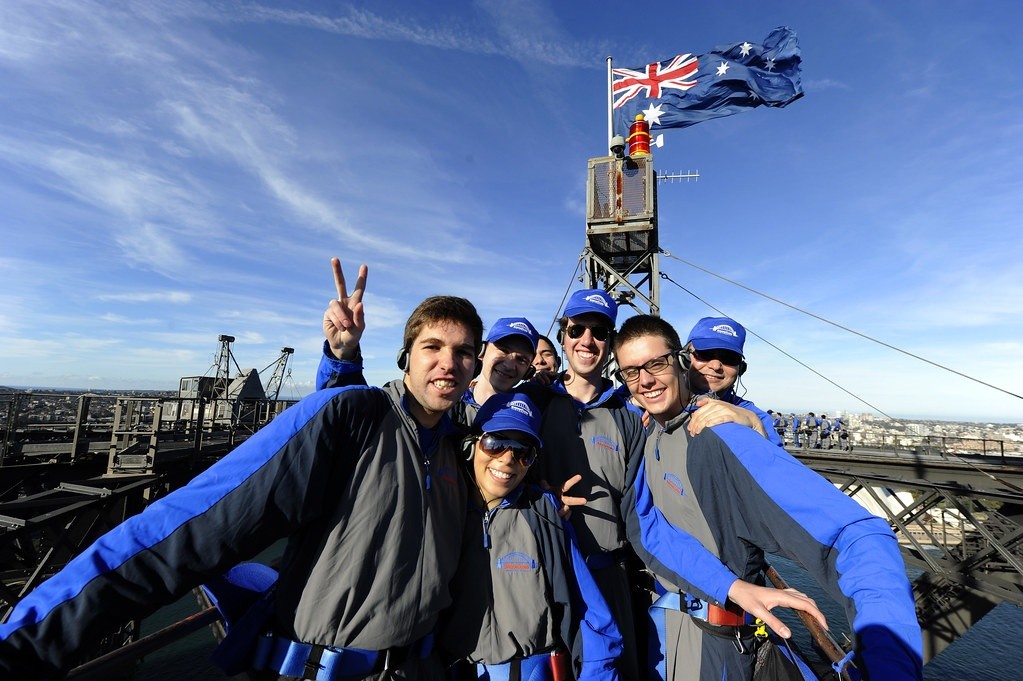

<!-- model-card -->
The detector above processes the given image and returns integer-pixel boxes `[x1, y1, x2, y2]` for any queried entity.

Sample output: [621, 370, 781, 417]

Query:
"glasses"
[473, 431, 537, 469]
[565, 325, 612, 341]
[615, 350, 680, 382]
[689, 348, 742, 366]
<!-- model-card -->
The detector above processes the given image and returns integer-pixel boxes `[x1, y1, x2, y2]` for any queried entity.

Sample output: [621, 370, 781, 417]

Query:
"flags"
[611, 26, 805, 143]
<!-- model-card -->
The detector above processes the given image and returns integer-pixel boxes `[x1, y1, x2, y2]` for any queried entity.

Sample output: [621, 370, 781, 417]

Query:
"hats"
[486, 317, 539, 356]
[685, 317, 746, 359]
[563, 290, 618, 329]
[470, 393, 543, 449]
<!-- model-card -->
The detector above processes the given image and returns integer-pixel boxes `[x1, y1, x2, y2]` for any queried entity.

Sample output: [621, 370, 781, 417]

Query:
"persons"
[315, 257, 623, 681]
[766, 410, 847, 448]
[614, 315, 924, 681]
[0, 297, 587, 681]
[514, 290, 829, 681]
[447, 317, 656, 473]
[530, 334, 562, 374]
[683, 317, 783, 438]
